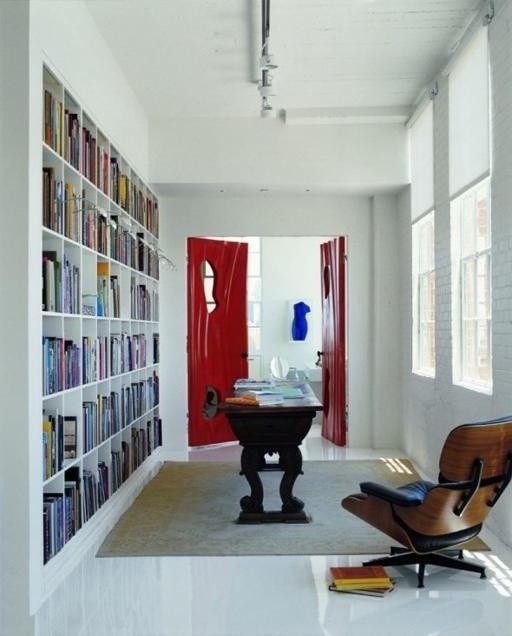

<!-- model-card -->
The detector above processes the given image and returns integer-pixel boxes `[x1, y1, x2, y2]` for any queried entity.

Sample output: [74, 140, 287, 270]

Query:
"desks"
[228, 383, 323, 526]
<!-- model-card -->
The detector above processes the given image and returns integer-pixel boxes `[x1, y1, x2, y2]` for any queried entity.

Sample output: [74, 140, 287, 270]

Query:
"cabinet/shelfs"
[42, 54, 162, 588]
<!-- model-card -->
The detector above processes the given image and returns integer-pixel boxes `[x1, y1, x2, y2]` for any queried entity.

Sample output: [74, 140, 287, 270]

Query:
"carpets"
[98, 457, 492, 558]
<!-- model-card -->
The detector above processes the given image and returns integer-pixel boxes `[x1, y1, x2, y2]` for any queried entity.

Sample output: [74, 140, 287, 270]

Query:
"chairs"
[342, 416, 512, 588]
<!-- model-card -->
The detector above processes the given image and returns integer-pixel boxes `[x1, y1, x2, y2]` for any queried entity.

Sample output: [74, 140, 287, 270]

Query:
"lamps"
[255, 29, 278, 122]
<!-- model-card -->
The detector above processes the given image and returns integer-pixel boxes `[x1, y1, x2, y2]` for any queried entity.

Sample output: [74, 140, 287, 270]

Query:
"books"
[42, 91, 158, 320]
[328, 567, 397, 598]
[226, 378, 304, 407]
[42, 333, 163, 563]
